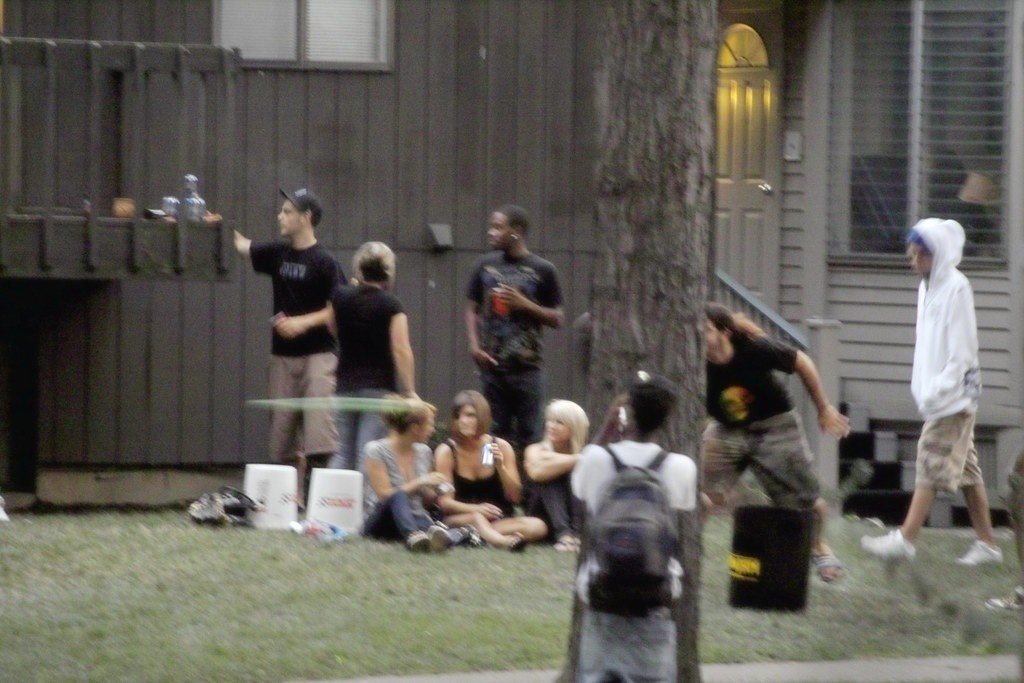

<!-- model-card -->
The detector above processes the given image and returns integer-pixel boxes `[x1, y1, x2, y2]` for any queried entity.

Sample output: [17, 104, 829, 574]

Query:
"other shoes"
[406, 524, 449, 552]
[505, 534, 532, 554]
[554, 533, 582, 554]
[812, 546, 842, 582]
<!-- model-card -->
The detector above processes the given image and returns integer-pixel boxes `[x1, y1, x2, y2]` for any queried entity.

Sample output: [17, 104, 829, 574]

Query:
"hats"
[278, 185, 323, 226]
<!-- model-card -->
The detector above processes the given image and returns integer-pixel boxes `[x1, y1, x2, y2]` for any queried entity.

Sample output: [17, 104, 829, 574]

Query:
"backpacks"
[576, 443, 683, 617]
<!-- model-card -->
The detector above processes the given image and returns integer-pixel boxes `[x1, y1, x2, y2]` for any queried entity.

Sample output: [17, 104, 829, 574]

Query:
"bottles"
[480, 443, 496, 469]
[178, 174, 207, 222]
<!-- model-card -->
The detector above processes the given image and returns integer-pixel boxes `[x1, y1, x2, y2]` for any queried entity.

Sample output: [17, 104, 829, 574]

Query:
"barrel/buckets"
[728, 503, 812, 612]
[306, 468, 366, 536]
[242, 463, 299, 531]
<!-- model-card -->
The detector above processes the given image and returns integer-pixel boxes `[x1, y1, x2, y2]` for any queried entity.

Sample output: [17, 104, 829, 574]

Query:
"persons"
[694, 302, 851, 585]
[861, 217, 1002, 566]
[570, 374, 698, 683]
[202, 185, 347, 513]
[985, 448, 1024, 611]
[326, 242, 420, 484]
[358, 390, 591, 556]
[465, 204, 565, 447]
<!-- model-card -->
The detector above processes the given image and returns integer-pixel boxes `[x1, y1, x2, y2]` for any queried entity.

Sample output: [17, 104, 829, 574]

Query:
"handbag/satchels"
[190, 487, 260, 525]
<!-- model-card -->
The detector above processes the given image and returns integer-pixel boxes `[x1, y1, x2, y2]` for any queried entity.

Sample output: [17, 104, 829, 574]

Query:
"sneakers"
[986, 585, 1022, 613]
[860, 529, 919, 562]
[955, 539, 1004, 568]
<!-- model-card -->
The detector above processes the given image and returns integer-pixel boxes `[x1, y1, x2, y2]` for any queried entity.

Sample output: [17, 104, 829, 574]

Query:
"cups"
[493, 287, 511, 315]
